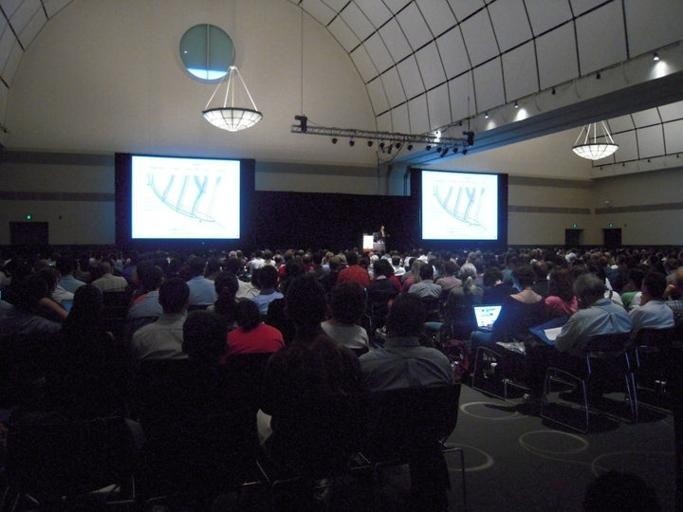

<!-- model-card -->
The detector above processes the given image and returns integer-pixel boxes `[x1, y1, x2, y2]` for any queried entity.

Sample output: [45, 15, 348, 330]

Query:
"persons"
[1, 246, 682, 511]
[370, 224, 391, 238]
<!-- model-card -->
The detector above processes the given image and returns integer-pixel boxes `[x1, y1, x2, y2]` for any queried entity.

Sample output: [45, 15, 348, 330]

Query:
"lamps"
[570, 120, 622, 161]
[202, 0, 263, 133]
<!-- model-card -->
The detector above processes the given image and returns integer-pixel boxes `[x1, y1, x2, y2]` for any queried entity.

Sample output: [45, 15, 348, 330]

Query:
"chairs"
[453, 271, 682, 433]
[0, 269, 466, 511]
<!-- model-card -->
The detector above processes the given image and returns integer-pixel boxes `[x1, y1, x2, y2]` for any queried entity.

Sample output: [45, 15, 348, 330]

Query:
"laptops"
[471, 304, 503, 330]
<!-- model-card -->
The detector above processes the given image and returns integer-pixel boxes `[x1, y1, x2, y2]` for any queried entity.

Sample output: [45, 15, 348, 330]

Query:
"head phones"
[577, 286, 614, 307]
